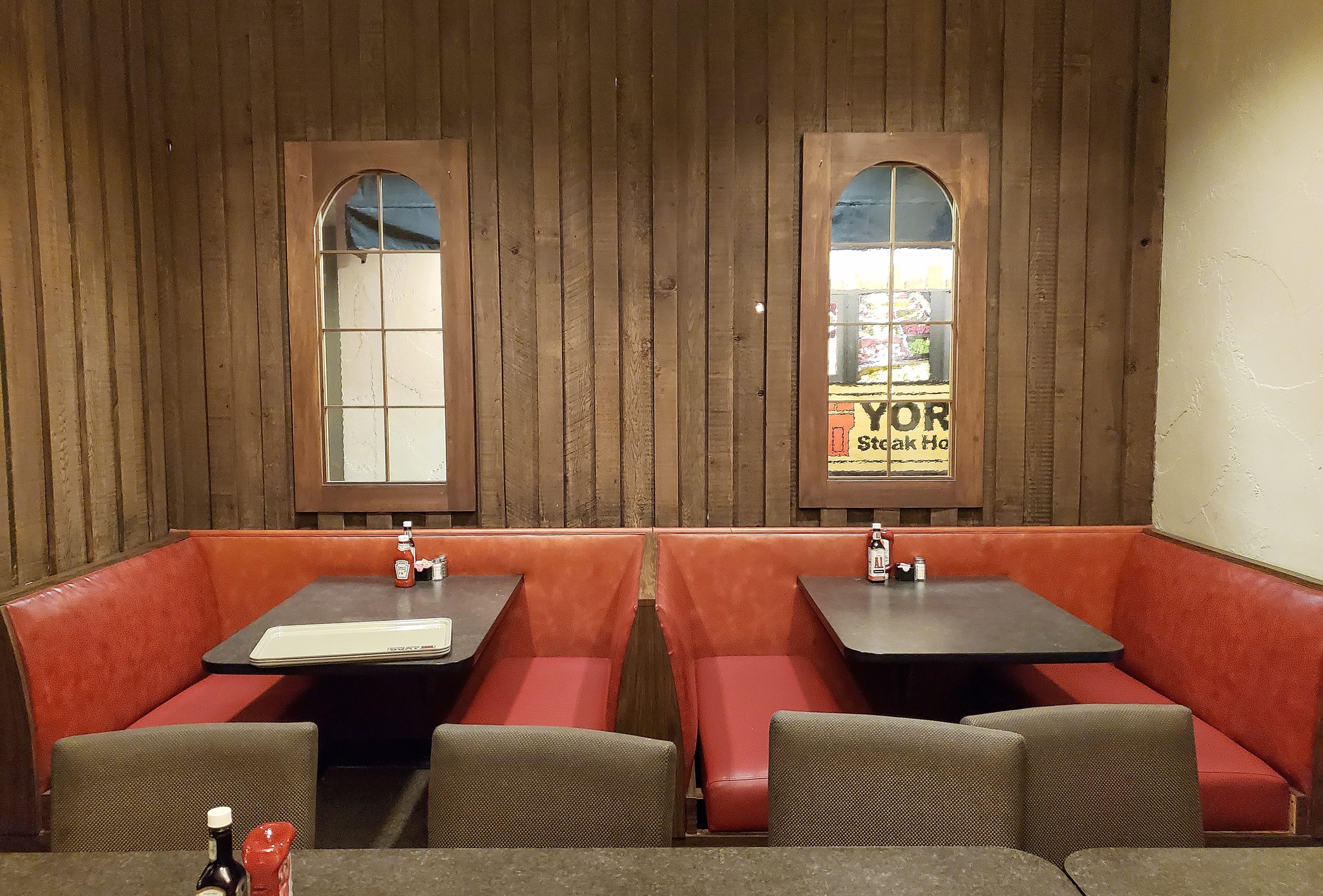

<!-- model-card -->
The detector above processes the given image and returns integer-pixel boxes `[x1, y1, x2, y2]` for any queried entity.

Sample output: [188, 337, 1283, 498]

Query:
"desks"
[1064, 847, 1323, 896]
[199, 573, 523, 772]
[0, 848, 1083, 896]
[798, 574, 1124, 720]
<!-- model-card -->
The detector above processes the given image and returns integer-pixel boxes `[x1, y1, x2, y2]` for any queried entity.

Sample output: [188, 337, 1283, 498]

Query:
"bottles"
[913, 556, 926, 581]
[403, 521, 416, 564]
[394, 535, 415, 588]
[438, 555, 447, 578]
[241, 822, 296, 896]
[867, 523, 886, 584]
[431, 557, 443, 580]
[196, 807, 248, 896]
[866, 529, 894, 580]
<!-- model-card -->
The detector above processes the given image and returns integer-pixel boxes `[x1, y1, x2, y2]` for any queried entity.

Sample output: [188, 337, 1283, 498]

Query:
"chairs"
[959, 705, 1205, 869]
[768, 707, 1026, 851]
[47, 722, 318, 849]
[427, 724, 678, 848]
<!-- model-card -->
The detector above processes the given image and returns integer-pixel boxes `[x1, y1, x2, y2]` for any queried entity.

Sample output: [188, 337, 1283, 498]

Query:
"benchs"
[1, 531, 317, 849]
[443, 537, 872, 847]
[1002, 529, 1323, 844]
